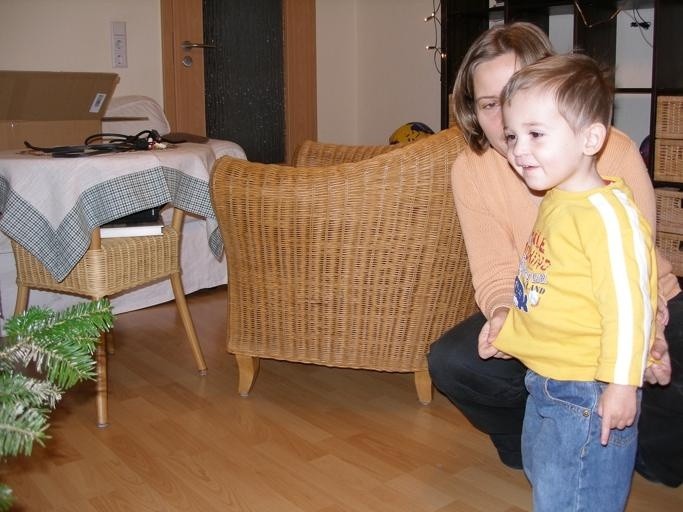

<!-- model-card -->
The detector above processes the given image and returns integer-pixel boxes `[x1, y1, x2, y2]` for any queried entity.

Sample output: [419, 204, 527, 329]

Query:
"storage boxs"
[1, 70, 119, 157]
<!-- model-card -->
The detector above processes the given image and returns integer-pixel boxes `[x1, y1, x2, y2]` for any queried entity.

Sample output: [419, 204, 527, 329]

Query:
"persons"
[424, 22, 683, 488]
[491, 50, 664, 511]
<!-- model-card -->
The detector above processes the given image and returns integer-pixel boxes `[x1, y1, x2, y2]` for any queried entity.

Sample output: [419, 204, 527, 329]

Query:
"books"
[99, 215, 164, 240]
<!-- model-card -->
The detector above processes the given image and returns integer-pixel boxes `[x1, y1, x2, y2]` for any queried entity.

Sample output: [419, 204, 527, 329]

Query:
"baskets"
[656, 96, 683, 139]
[656, 231, 683, 277]
[654, 187, 682, 235]
[654, 139, 683, 183]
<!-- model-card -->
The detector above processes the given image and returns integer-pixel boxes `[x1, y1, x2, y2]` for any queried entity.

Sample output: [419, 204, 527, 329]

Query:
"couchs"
[209, 123, 494, 404]
[0, 95, 249, 334]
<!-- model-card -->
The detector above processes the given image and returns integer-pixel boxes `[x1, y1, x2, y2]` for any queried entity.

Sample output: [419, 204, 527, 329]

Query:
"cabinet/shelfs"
[438, 1, 683, 213]
